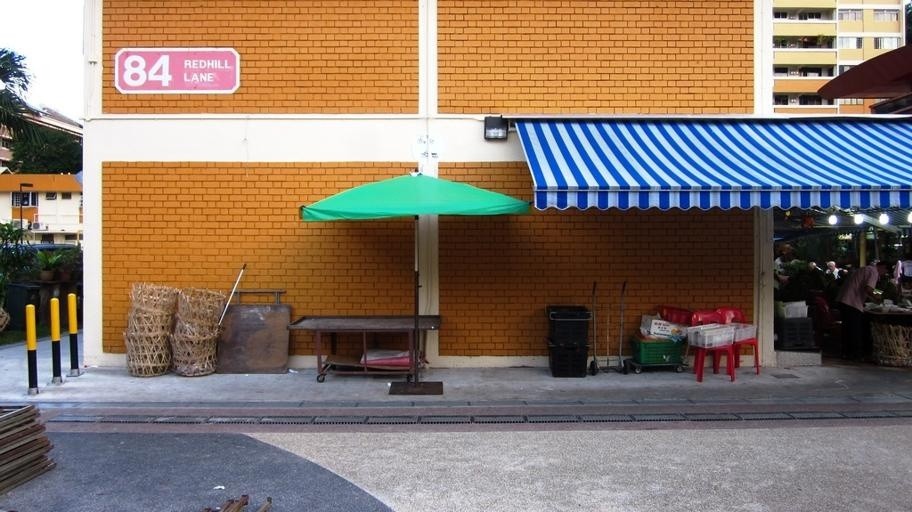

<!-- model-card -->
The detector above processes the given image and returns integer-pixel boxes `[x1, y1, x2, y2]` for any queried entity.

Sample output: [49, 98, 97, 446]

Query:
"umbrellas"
[302, 169, 531, 388]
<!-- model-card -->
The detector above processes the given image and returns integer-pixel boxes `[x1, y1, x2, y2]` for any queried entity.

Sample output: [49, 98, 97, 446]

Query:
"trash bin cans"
[7, 283, 41, 331]
[545, 305, 592, 378]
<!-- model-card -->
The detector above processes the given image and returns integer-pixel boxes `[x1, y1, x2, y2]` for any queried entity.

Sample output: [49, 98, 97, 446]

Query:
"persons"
[825, 261, 839, 279]
[892, 254, 912, 287]
[809, 261, 823, 272]
[836, 260, 890, 361]
[773, 251, 789, 301]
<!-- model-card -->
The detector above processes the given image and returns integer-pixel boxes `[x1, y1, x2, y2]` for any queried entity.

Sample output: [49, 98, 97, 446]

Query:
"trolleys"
[590, 276, 631, 374]
[285, 314, 443, 385]
[628, 324, 691, 378]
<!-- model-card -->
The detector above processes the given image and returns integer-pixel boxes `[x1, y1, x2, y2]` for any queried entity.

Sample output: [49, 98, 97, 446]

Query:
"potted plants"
[37, 249, 63, 281]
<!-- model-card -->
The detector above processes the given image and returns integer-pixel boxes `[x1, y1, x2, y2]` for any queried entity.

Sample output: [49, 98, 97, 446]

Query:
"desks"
[863, 310, 912, 327]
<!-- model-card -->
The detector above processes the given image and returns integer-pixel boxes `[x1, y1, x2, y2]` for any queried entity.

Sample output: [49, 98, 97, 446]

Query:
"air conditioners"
[32, 221, 46, 231]
[12, 219, 29, 231]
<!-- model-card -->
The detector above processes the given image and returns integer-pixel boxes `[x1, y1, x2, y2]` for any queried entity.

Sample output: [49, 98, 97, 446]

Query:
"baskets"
[687, 323, 756, 349]
[122, 283, 227, 378]
[874, 324, 911, 368]
[633, 334, 688, 364]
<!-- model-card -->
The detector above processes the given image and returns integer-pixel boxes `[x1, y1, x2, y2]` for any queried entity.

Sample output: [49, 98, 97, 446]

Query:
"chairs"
[813, 293, 843, 345]
[690, 310, 736, 382]
[712, 307, 760, 375]
